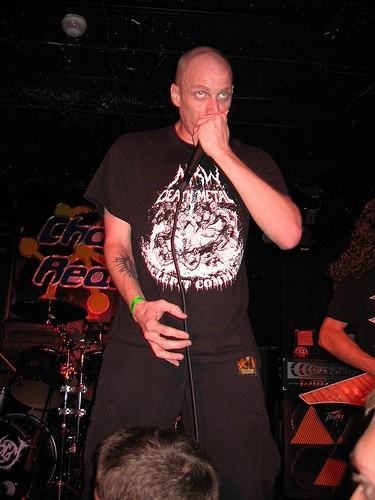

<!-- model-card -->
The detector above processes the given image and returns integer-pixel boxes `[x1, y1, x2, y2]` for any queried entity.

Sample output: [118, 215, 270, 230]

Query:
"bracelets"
[129, 295, 146, 316]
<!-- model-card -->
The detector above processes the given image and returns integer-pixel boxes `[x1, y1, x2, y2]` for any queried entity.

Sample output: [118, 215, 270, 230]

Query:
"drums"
[10, 345, 67, 411]
[75, 349, 104, 403]
[1, 413, 59, 500]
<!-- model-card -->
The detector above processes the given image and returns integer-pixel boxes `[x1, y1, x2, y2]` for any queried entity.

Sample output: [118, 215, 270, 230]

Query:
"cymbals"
[9, 299, 89, 324]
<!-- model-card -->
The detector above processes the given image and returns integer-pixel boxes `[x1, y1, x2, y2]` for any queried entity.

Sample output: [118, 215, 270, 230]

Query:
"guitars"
[298, 372, 375, 421]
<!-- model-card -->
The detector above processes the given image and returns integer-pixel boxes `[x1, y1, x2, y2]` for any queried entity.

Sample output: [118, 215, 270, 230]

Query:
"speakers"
[276, 394, 375, 500]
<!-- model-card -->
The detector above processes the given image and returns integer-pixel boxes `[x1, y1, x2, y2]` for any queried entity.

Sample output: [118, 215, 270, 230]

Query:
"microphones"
[180, 141, 204, 192]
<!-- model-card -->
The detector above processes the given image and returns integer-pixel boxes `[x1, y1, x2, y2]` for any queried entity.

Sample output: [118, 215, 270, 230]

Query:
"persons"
[92, 425, 220, 500]
[86, 46, 302, 500]
[349, 390, 375, 500]
[317, 201, 375, 374]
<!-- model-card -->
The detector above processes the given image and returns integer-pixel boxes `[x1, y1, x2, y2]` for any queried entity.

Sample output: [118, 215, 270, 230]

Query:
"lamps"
[62, 14, 88, 38]
